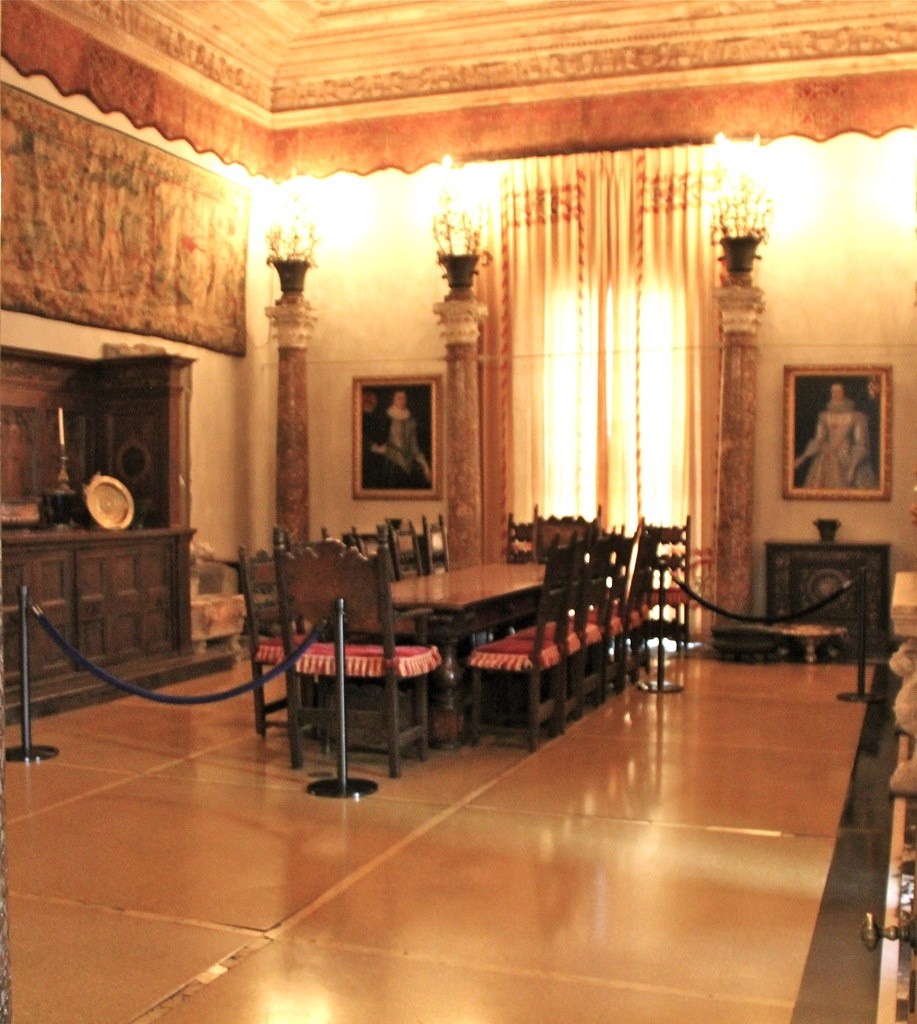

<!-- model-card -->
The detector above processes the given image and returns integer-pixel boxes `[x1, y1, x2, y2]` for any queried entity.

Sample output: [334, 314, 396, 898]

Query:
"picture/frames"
[352, 374, 444, 501]
[783, 363, 892, 502]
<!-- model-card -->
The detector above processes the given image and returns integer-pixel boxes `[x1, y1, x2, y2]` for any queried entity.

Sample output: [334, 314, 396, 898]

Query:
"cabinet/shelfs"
[0, 342, 200, 696]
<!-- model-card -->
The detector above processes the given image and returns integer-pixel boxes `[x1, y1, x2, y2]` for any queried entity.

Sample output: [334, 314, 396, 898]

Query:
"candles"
[57, 407, 67, 456]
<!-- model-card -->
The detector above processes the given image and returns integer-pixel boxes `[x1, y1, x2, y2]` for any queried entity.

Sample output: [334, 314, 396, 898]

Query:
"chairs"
[282, 526, 378, 645]
[272, 523, 441, 779]
[237, 544, 331, 739]
[351, 525, 392, 581]
[534, 505, 604, 566]
[467, 531, 579, 752]
[421, 514, 455, 576]
[639, 515, 692, 650]
[556, 524, 626, 703]
[589, 519, 645, 693]
[505, 529, 595, 736]
[385, 518, 422, 581]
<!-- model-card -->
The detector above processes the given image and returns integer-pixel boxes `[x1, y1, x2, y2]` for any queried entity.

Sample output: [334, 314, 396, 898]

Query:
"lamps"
[266, 167, 319, 306]
[708, 132, 773, 287]
[431, 153, 484, 300]
